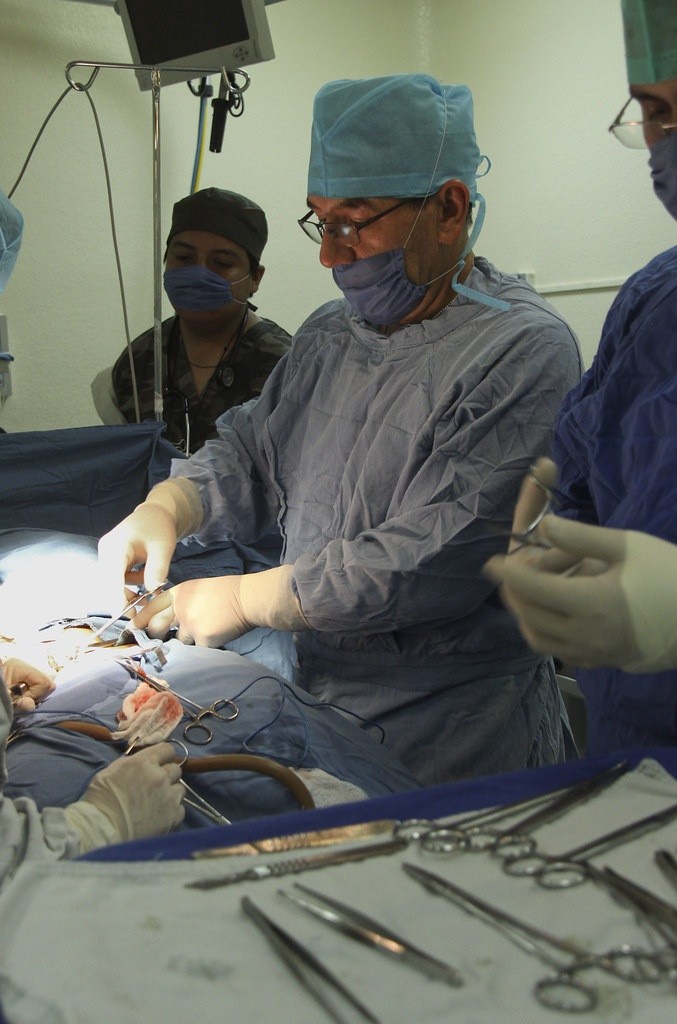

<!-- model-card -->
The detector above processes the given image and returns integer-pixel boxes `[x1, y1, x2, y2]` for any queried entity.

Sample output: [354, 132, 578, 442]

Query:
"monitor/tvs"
[118, 0, 276, 92]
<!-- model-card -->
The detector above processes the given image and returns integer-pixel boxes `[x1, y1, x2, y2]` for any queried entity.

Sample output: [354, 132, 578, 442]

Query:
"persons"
[482, 0, 677, 785]
[0, 189, 190, 879]
[97, 76, 584, 786]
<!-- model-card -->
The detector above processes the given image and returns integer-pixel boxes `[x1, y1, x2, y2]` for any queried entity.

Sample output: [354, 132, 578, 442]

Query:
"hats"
[170, 187, 268, 261]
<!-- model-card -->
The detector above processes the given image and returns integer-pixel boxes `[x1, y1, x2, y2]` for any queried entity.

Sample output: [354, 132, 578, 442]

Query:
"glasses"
[609, 97, 677, 149]
[297, 198, 414, 247]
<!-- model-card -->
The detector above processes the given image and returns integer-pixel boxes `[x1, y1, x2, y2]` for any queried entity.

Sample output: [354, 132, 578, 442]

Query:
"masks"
[162, 266, 251, 313]
[648, 132, 677, 222]
[332, 247, 429, 326]
